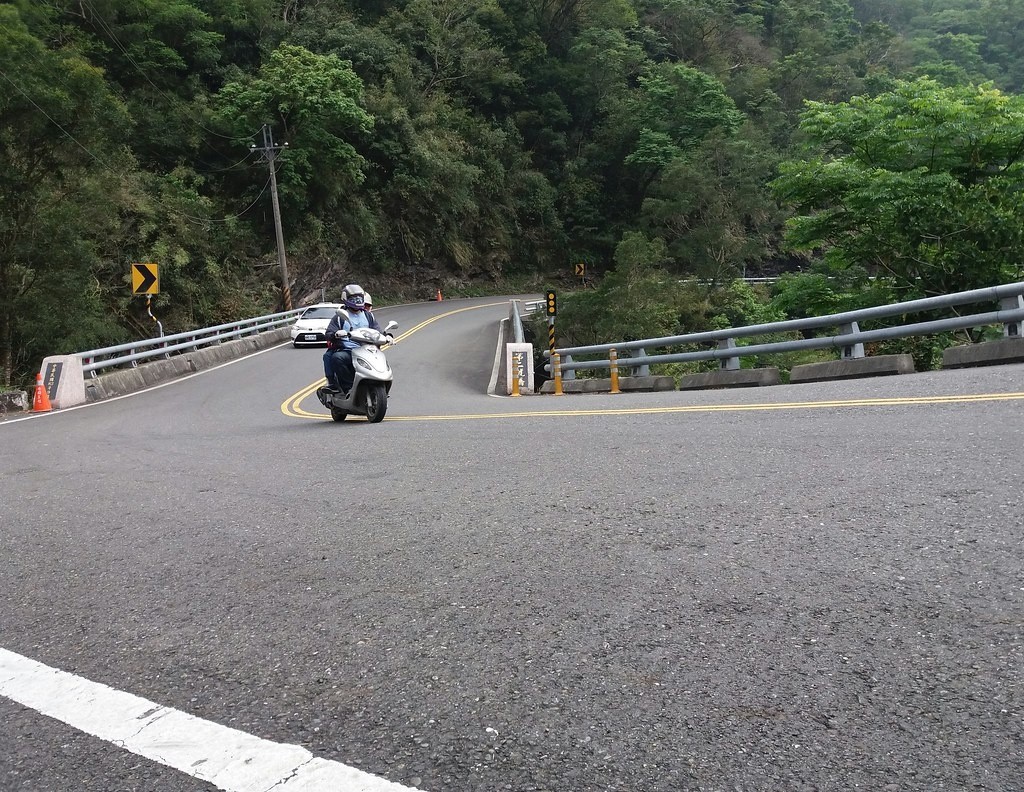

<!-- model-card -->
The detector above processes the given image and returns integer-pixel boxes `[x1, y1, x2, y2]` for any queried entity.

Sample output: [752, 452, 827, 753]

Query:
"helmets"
[364, 291, 373, 311]
[341, 284, 366, 310]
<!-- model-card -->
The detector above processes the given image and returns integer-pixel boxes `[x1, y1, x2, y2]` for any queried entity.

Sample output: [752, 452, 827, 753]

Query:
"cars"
[290, 302, 345, 349]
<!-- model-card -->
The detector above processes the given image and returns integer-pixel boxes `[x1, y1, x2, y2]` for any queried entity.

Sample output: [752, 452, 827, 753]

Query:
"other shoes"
[322, 384, 340, 394]
[345, 391, 350, 399]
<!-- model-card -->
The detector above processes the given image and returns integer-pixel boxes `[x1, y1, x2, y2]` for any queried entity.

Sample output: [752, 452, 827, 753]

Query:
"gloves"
[335, 330, 347, 338]
[386, 335, 394, 346]
[327, 340, 336, 349]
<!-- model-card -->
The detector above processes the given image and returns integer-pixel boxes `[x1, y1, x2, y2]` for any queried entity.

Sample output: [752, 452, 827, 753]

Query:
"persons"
[320, 283, 395, 399]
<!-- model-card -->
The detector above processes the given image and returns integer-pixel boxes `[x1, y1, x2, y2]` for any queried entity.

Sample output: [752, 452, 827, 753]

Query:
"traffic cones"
[28, 373, 55, 412]
[437, 288, 442, 302]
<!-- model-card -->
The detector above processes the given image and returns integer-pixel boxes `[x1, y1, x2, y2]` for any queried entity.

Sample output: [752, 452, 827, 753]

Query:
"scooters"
[317, 309, 399, 424]
